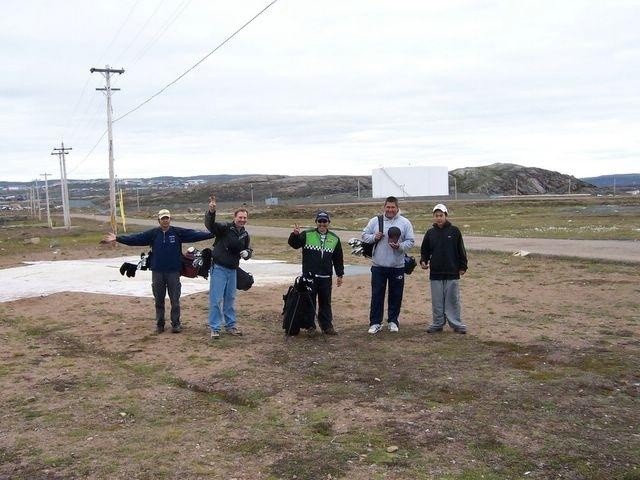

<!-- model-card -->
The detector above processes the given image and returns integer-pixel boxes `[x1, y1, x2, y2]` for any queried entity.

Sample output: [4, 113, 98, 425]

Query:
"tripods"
[285, 292, 327, 343]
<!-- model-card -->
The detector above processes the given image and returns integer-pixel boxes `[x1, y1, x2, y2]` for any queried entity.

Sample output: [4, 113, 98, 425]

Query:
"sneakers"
[388, 322, 398, 332]
[211, 330, 220, 339]
[154, 323, 183, 333]
[323, 327, 338, 335]
[368, 324, 382, 334]
[454, 327, 467, 334]
[225, 328, 242, 336]
[427, 326, 443, 333]
[306, 327, 316, 337]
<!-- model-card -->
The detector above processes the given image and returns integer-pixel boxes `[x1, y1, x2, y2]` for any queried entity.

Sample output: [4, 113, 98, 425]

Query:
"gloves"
[240, 250, 249, 258]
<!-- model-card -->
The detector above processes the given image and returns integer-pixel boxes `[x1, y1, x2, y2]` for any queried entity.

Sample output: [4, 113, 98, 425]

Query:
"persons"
[104, 209, 218, 335]
[419, 204, 468, 335]
[204, 195, 253, 339]
[288, 211, 345, 340]
[361, 196, 415, 336]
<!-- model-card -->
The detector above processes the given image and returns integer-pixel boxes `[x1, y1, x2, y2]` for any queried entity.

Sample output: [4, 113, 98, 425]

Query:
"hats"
[315, 213, 331, 222]
[388, 227, 401, 245]
[158, 209, 171, 220]
[433, 204, 448, 216]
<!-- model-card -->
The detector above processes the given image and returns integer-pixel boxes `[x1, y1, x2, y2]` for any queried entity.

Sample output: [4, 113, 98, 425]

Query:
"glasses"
[318, 219, 328, 223]
